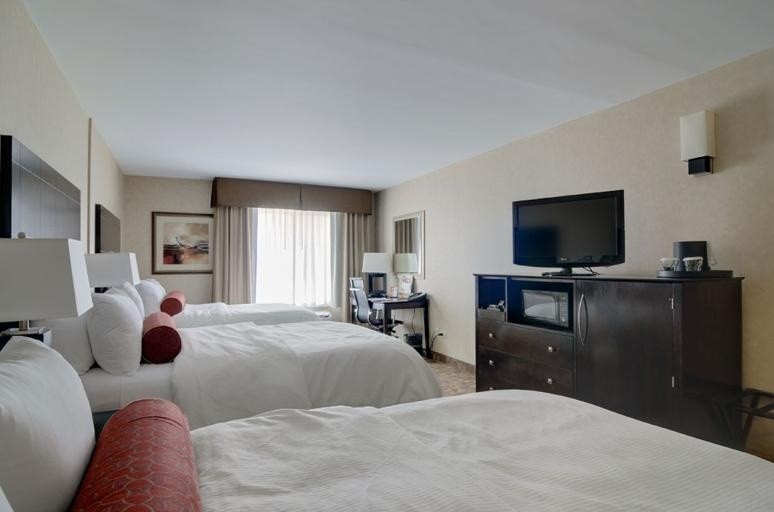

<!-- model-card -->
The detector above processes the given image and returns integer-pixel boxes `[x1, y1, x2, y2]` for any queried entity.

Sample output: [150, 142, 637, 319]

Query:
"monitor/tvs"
[512, 189, 625, 276]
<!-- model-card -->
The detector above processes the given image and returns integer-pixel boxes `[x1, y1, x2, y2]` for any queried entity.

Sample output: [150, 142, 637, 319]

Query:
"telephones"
[408, 292, 426, 301]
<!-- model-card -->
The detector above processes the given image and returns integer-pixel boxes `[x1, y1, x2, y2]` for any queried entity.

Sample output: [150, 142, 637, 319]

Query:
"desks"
[348, 295, 431, 359]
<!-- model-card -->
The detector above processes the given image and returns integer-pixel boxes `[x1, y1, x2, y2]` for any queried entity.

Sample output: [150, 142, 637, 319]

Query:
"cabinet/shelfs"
[573, 275, 744, 450]
[473, 273, 575, 398]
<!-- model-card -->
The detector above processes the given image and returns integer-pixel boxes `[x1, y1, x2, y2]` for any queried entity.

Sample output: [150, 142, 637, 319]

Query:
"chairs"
[349, 277, 404, 339]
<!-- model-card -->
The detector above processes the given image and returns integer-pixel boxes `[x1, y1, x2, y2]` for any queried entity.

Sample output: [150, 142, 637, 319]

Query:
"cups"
[658, 255, 703, 272]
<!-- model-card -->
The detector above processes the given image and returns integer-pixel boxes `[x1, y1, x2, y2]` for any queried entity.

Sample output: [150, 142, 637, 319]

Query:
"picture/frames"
[151, 211, 215, 274]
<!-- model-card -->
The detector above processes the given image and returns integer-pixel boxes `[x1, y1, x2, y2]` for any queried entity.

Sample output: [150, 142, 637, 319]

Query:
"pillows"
[30, 314, 95, 377]
[0, 332, 101, 510]
[0, 484, 13, 511]
[146, 278, 167, 298]
[87, 289, 143, 376]
[160, 291, 186, 316]
[66, 398, 198, 512]
[141, 312, 181, 362]
[135, 280, 164, 314]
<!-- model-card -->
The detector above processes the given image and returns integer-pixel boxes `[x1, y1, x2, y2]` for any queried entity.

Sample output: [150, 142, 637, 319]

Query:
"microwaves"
[519, 288, 570, 328]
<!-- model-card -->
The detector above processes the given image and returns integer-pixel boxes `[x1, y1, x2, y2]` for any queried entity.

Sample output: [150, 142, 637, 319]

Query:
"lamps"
[361, 252, 388, 297]
[393, 252, 419, 274]
[391, 208, 426, 278]
[85, 251, 142, 293]
[0, 233, 94, 342]
[679, 109, 716, 175]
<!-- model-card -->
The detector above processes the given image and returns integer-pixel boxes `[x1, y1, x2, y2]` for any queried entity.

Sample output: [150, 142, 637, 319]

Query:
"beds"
[28, 311, 444, 431]
[75, 384, 773, 512]
[173, 303, 322, 328]
